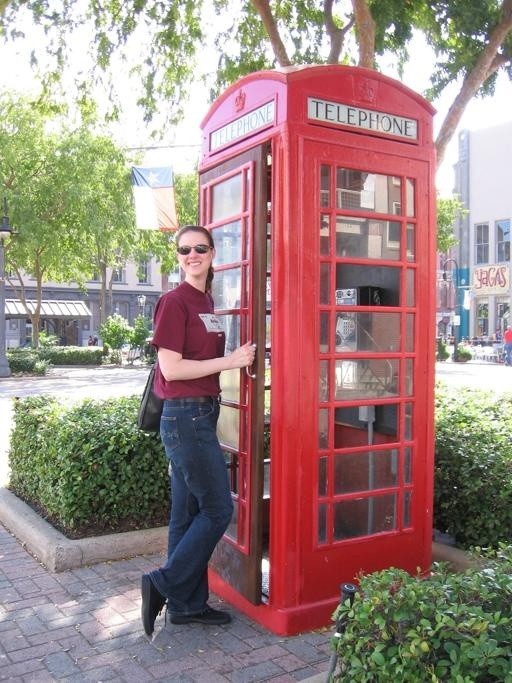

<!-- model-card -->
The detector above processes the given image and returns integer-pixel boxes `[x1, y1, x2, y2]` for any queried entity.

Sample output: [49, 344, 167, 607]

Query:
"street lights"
[443, 259, 458, 314]
[0, 217, 14, 376]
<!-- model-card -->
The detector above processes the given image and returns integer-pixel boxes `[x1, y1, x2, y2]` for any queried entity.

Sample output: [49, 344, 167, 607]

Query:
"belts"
[165, 394, 221, 404]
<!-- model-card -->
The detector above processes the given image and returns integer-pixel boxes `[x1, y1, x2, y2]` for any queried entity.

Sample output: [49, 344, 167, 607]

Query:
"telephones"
[316, 286, 371, 389]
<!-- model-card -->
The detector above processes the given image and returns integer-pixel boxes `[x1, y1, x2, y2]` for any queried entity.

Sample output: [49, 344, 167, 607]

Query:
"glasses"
[175, 244, 213, 255]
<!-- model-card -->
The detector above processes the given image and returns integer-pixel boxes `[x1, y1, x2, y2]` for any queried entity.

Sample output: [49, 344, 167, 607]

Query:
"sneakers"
[169, 604, 232, 624]
[141, 572, 166, 636]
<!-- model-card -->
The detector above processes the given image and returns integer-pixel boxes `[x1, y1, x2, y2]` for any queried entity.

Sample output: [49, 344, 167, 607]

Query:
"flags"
[131, 163, 179, 232]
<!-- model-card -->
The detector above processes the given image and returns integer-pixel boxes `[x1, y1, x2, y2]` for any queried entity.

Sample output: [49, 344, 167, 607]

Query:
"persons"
[138, 225, 259, 636]
[88, 335, 93, 345]
[503, 324, 512, 367]
[93, 334, 99, 345]
[496, 329, 504, 363]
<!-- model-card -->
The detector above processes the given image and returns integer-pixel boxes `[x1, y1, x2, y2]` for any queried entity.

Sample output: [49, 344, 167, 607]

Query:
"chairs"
[458, 342, 505, 362]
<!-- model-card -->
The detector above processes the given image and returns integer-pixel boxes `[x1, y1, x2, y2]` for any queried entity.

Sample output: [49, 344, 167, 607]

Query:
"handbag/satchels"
[138, 361, 163, 430]
